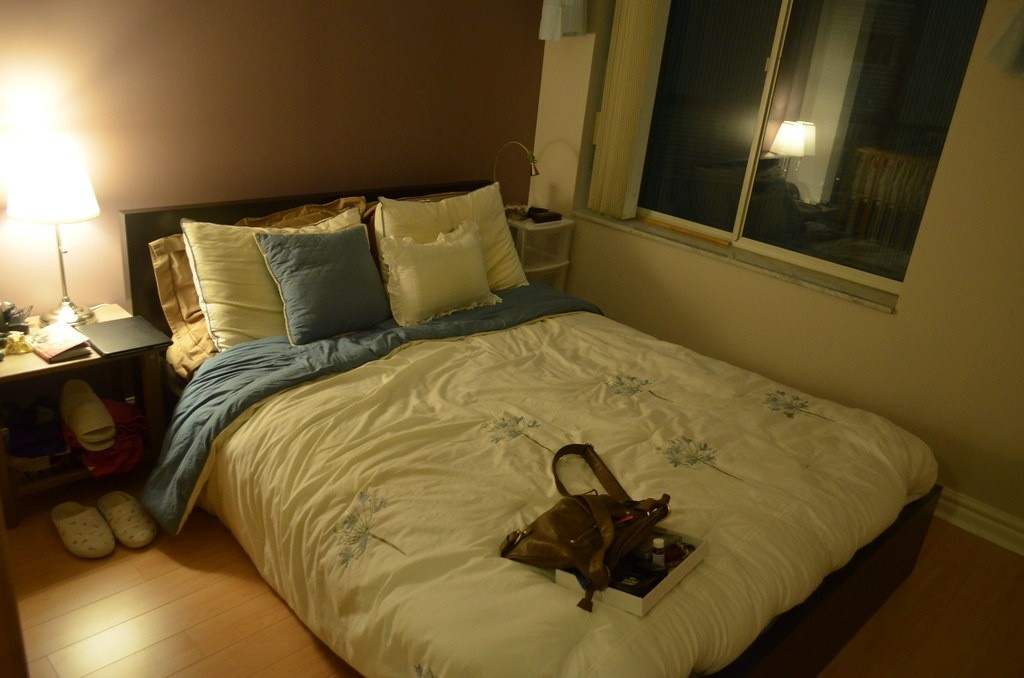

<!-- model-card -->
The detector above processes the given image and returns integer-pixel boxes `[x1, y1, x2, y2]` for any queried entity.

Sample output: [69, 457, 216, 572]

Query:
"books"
[27, 322, 90, 363]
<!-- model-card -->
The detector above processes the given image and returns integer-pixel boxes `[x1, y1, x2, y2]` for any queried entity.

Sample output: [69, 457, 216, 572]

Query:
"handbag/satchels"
[500, 443, 671, 612]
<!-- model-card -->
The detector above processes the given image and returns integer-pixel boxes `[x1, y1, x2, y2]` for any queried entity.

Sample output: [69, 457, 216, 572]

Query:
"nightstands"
[796, 198, 840, 245]
[0, 304, 168, 530]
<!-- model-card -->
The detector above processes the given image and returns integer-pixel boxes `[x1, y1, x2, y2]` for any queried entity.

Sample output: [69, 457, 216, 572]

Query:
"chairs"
[808, 148, 938, 279]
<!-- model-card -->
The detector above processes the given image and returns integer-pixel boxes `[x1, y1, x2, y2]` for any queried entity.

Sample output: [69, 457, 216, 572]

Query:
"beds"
[115, 182, 938, 678]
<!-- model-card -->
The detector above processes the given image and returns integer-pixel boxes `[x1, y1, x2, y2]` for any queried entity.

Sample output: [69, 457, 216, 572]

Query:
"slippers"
[59, 378, 116, 452]
[97, 490, 157, 548]
[51, 502, 114, 558]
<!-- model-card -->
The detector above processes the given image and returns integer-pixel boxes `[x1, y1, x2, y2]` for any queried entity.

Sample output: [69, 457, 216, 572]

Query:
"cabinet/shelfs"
[506, 204, 578, 295]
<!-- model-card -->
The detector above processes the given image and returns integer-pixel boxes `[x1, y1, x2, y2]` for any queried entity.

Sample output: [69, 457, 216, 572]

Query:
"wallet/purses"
[611, 556, 669, 597]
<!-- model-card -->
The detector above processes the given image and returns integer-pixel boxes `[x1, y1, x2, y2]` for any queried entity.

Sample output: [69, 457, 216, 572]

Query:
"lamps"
[770, 119, 817, 182]
[491, 142, 541, 184]
[7, 130, 101, 330]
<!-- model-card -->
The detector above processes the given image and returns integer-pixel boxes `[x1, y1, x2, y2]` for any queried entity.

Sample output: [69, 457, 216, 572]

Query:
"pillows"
[180, 207, 361, 354]
[374, 182, 529, 294]
[378, 219, 505, 328]
[252, 223, 394, 348]
[148, 196, 367, 374]
